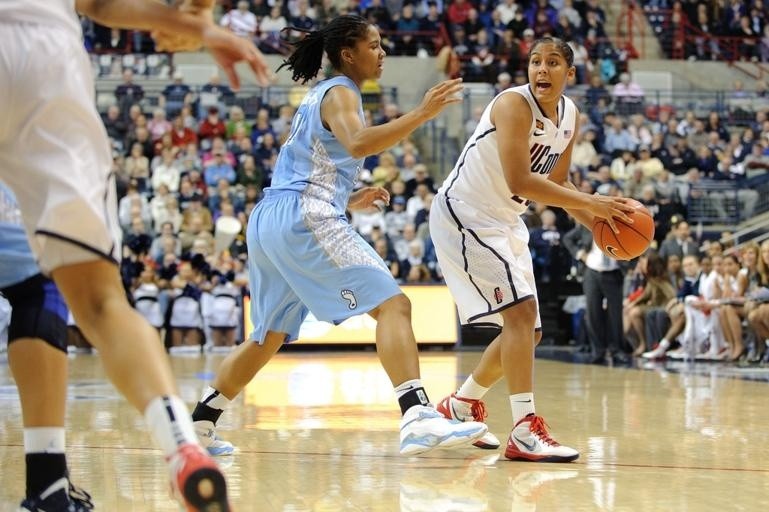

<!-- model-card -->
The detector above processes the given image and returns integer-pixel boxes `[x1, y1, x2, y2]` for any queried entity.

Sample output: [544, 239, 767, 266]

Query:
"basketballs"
[593, 198, 655, 260]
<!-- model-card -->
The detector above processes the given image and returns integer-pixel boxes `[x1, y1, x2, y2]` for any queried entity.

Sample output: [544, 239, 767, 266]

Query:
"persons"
[193, 14, 487, 458]
[69, 1, 768, 364]
[427, 37, 636, 463]
[1, 2, 274, 512]
[0, 178, 99, 512]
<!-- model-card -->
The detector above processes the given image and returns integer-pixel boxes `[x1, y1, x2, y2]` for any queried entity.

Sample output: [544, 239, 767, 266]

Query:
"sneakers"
[504, 413, 579, 462]
[195, 419, 236, 457]
[21, 477, 96, 511]
[437, 394, 501, 450]
[167, 444, 232, 512]
[397, 402, 488, 459]
[612, 352, 631, 365]
[642, 347, 768, 364]
[585, 355, 606, 364]
